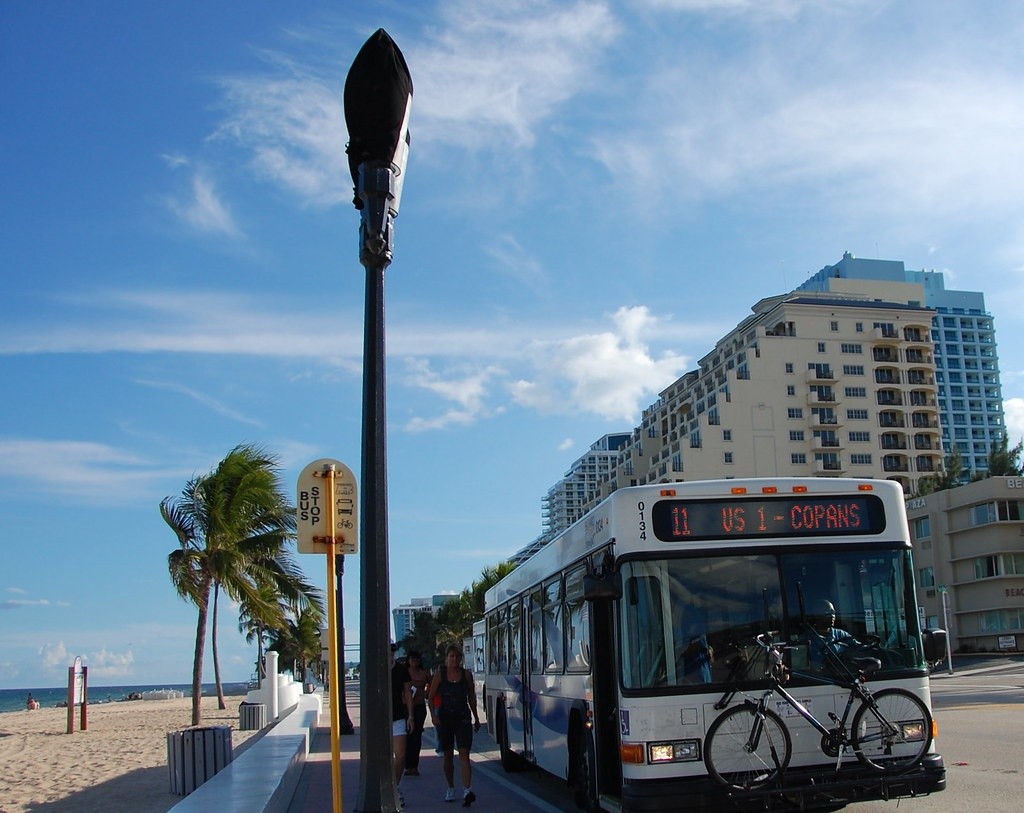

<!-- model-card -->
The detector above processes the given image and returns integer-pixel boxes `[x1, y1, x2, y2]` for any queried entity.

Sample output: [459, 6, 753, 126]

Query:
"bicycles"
[704, 625, 935, 795]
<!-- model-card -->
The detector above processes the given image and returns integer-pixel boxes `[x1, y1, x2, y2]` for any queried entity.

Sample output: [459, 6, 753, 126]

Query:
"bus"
[484, 474, 947, 813]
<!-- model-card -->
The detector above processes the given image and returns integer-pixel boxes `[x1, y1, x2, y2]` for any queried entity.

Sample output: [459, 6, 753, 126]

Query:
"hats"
[390, 638, 395, 644]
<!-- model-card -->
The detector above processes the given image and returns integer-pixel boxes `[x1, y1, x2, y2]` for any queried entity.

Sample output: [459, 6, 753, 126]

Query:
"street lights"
[343, 26, 413, 812]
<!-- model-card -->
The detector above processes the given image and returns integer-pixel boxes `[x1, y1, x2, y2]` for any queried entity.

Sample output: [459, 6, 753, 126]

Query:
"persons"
[680, 603, 714, 684]
[390, 637, 481, 813]
[791, 598, 868, 673]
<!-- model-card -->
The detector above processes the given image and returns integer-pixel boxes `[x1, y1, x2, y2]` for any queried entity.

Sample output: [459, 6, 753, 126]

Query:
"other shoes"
[397, 785, 405, 806]
[404, 769, 420, 776]
[436, 752, 444, 758]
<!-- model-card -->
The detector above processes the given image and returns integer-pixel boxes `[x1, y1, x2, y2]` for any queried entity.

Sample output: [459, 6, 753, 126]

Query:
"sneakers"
[445, 788, 455, 801]
[463, 787, 475, 807]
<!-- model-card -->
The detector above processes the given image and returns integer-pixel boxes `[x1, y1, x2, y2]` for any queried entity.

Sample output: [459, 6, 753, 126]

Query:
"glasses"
[823, 608, 837, 617]
[409, 656, 420, 659]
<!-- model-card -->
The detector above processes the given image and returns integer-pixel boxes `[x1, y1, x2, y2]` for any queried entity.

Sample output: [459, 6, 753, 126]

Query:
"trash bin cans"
[239, 702, 267, 730]
[304, 683, 313, 693]
[165, 725, 233, 795]
